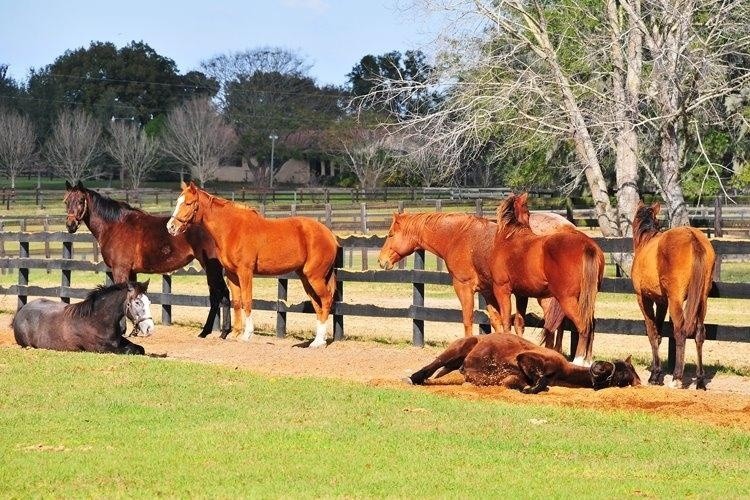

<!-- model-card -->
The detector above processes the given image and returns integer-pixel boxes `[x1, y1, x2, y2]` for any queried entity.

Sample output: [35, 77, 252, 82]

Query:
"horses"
[401, 331, 641, 395]
[377, 191, 606, 368]
[165, 179, 341, 351]
[63, 179, 233, 340]
[9, 277, 157, 356]
[630, 198, 717, 391]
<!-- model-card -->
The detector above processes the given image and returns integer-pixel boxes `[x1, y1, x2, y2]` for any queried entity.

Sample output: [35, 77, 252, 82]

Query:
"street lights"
[269, 128, 279, 190]
